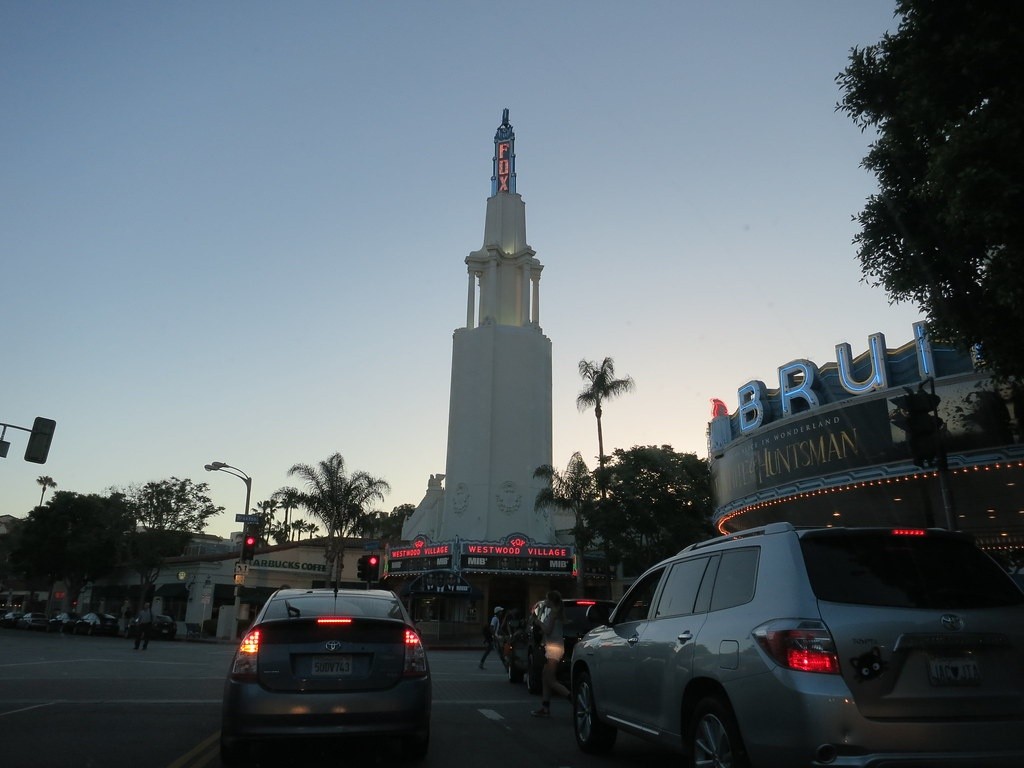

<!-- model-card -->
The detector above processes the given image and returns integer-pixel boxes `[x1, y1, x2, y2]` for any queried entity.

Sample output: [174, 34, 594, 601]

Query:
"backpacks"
[482, 616, 498, 646]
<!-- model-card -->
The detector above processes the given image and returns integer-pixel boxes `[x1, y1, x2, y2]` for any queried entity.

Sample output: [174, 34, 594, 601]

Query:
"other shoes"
[143, 647, 147, 650]
[133, 646, 139, 649]
[479, 663, 483, 668]
[530, 707, 551, 718]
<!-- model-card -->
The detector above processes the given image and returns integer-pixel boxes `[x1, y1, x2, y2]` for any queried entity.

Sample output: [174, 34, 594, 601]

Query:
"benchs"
[185, 622, 208, 639]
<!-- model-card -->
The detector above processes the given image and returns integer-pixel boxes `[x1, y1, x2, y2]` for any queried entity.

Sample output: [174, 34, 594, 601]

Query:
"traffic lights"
[357, 555, 369, 582]
[242, 524, 258, 561]
[367, 556, 379, 582]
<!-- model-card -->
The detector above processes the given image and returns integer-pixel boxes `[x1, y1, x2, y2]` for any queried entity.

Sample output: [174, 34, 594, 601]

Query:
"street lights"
[204, 461, 253, 641]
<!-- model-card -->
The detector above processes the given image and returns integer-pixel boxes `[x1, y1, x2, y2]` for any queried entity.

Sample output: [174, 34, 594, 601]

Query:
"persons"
[478, 607, 509, 670]
[124, 601, 153, 650]
[530, 590, 570, 719]
[421, 572, 460, 592]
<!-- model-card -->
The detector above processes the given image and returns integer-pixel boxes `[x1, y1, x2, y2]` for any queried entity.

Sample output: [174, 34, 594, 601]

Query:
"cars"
[124, 613, 178, 640]
[46, 611, 81, 634]
[0, 611, 25, 627]
[223, 587, 434, 762]
[73, 611, 120, 636]
[16, 612, 50, 631]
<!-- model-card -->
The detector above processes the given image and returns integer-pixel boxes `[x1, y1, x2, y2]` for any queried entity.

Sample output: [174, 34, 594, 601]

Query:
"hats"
[494, 606, 504, 613]
[144, 602, 150, 609]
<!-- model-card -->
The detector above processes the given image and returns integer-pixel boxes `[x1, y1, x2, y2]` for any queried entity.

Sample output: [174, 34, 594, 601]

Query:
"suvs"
[502, 599, 647, 693]
[568, 522, 1023, 768]
